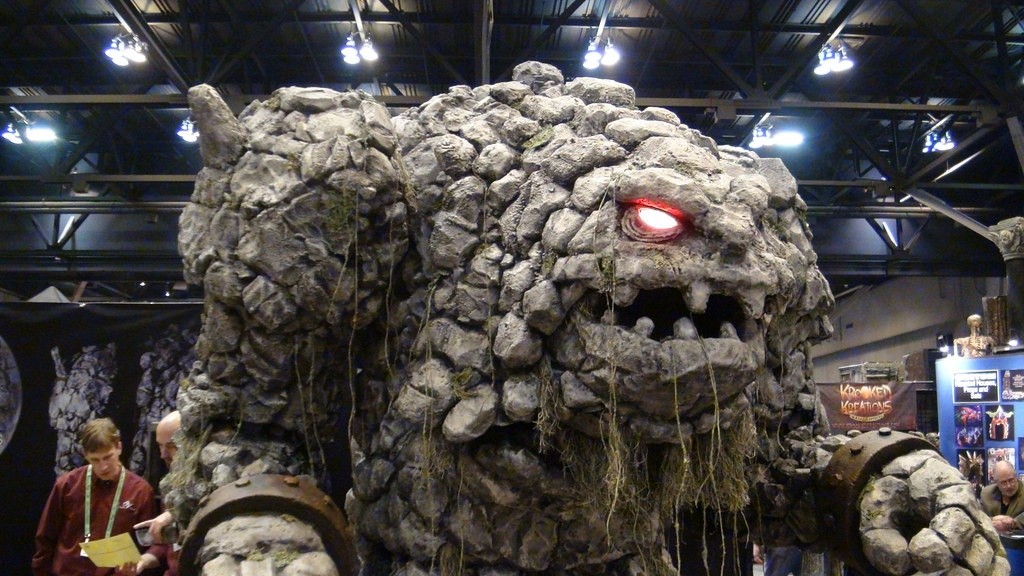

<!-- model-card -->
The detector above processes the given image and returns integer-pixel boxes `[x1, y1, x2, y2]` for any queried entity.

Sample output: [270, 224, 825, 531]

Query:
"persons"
[981, 460, 1024, 532]
[30, 417, 155, 576]
[116, 411, 180, 574]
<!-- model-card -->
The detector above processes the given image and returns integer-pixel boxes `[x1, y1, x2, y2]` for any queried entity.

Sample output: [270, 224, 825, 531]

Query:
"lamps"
[748, 124, 773, 149]
[2, 121, 23, 144]
[104, 27, 149, 66]
[176, 115, 200, 142]
[340, 28, 379, 65]
[583, 30, 620, 69]
[921, 130, 953, 153]
[813, 42, 853, 75]
